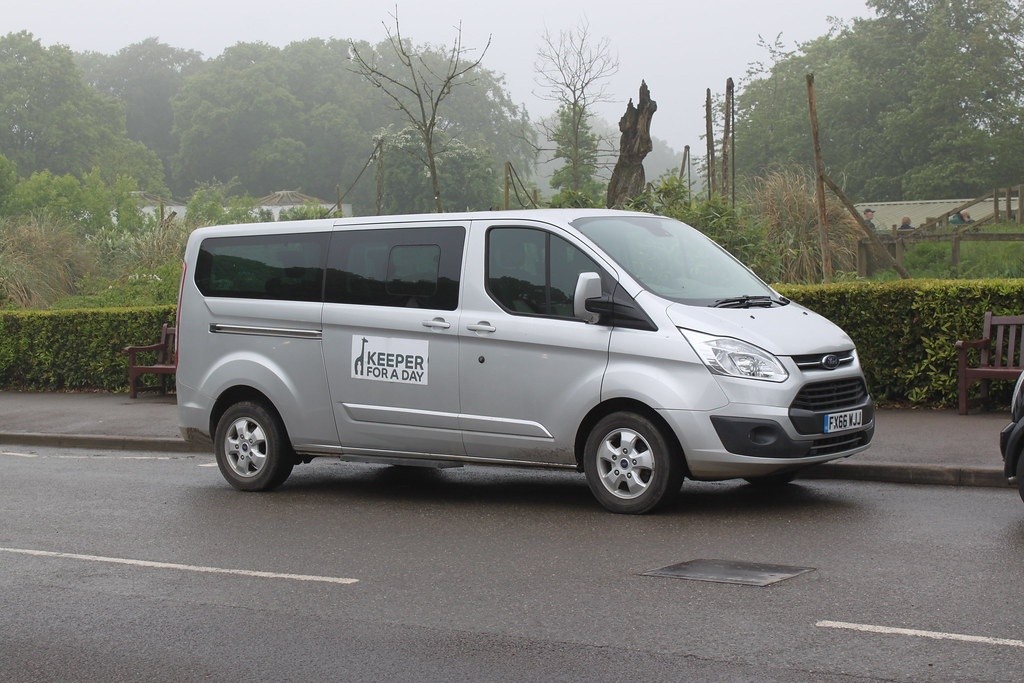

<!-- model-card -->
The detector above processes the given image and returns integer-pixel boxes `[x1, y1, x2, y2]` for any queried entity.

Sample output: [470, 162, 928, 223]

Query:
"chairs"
[500, 241, 545, 313]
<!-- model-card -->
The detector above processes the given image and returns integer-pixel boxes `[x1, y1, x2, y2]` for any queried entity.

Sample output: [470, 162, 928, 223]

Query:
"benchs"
[123, 323, 175, 399]
[955, 311, 1024, 415]
[267, 252, 341, 302]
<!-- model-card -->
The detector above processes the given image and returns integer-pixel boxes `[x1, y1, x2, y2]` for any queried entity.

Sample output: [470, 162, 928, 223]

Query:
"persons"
[898, 217, 916, 229]
[863, 208, 876, 230]
[949, 209, 975, 224]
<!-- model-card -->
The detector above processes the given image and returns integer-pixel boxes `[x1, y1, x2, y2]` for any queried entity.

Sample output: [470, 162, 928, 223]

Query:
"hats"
[863, 209, 875, 214]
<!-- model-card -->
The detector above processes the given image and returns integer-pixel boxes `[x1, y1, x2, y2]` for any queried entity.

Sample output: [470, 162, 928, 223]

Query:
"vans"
[174, 208, 876, 515]
